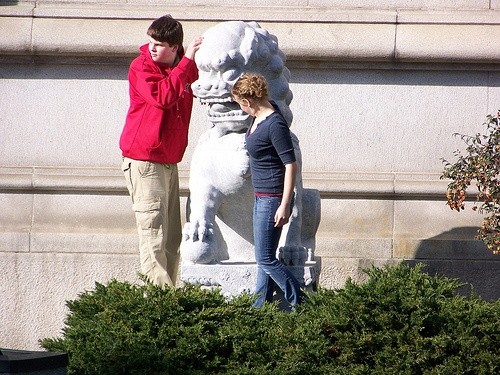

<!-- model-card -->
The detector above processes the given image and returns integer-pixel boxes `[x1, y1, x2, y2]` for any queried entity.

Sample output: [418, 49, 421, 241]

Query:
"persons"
[119, 14, 204, 289]
[232, 73, 306, 309]
